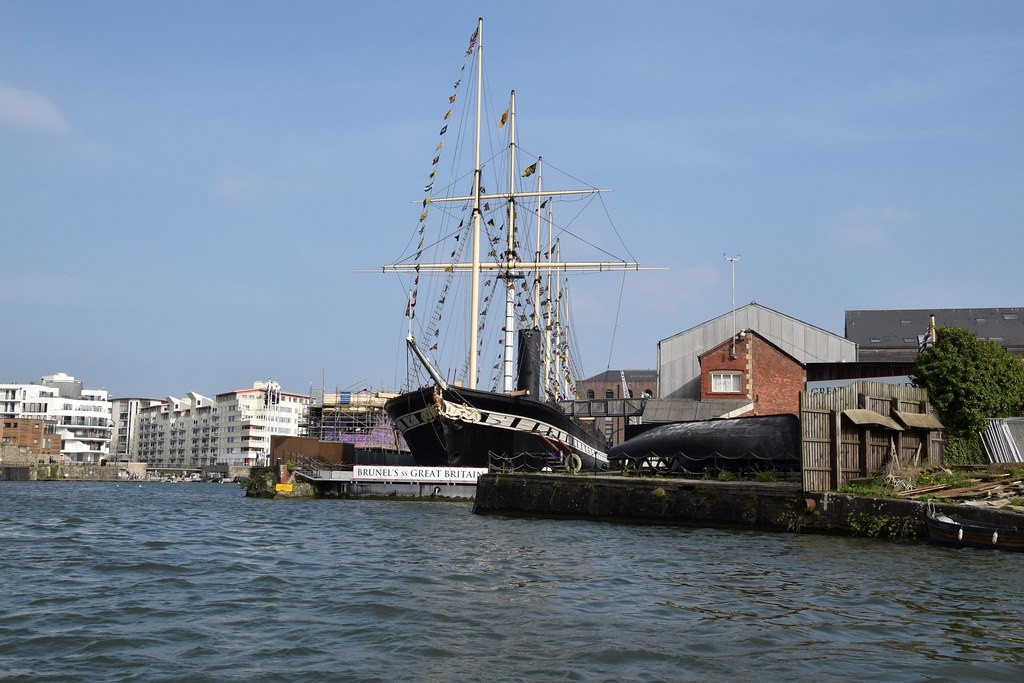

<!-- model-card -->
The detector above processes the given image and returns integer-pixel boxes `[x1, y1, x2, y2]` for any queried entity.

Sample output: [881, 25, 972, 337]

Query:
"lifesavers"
[565, 453, 582, 473]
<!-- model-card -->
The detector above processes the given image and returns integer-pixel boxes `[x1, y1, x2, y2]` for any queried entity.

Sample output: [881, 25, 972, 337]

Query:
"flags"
[535, 200, 548, 211]
[464, 25, 478, 55]
[544, 244, 556, 259]
[498, 107, 510, 128]
[521, 163, 536, 177]
[920, 322, 930, 350]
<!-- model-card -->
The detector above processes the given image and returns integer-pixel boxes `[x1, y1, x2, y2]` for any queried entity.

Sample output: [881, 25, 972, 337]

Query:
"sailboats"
[353, 17, 670, 467]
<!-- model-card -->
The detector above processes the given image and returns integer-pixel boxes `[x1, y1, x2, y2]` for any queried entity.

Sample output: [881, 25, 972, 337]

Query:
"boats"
[923, 503, 1024, 551]
